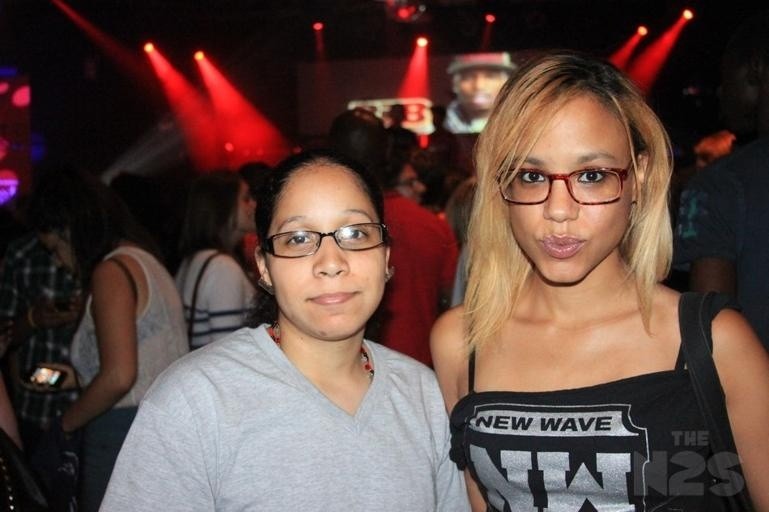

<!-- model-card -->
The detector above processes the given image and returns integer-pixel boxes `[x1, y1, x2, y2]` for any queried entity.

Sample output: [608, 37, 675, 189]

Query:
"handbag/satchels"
[30, 396, 82, 511]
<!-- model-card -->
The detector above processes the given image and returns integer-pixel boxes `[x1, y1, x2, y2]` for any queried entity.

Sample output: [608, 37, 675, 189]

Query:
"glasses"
[499, 158, 634, 205]
[261, 223, 389, 258]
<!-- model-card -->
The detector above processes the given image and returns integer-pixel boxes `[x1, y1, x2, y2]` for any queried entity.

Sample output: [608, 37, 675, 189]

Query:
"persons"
[0, 107, 769, 511]
[441, 52, 516, 134]
[429, 46, 768, 510]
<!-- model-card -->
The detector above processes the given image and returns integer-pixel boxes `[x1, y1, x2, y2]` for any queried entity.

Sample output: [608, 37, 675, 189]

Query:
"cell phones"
[24, 364, 68, 388]
